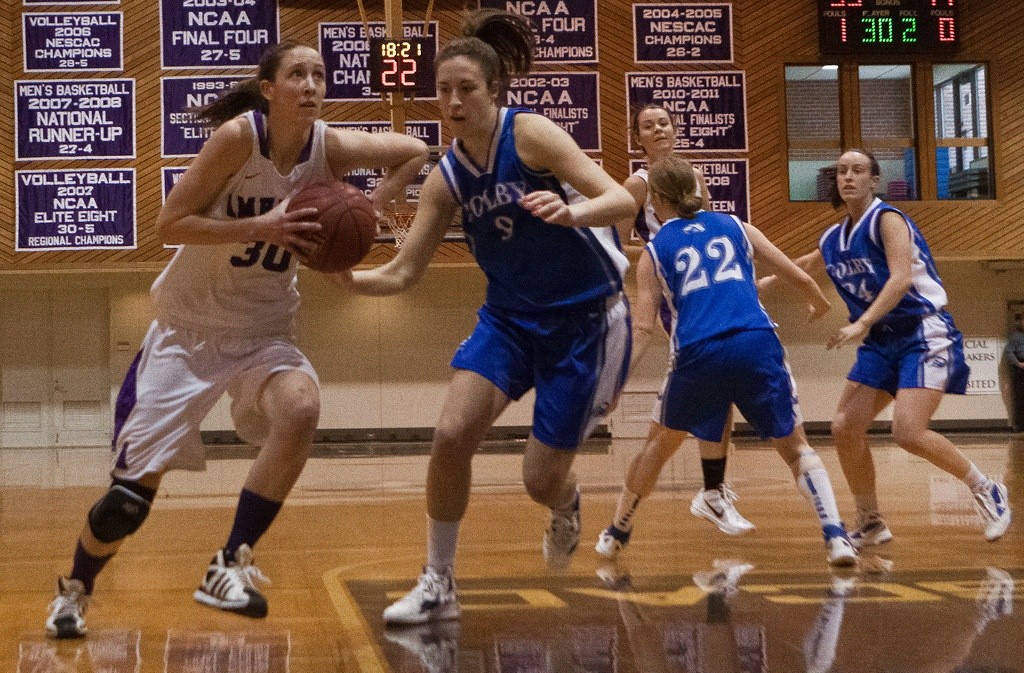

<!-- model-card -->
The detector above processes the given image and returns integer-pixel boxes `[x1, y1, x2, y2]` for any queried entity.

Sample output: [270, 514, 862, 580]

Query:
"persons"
[596, 156, 859, 566]
[757, 147, 1011, 545]
[45, 41, 430, 640]
[613, 104, 755, 536]
[329, 8, 637, 625]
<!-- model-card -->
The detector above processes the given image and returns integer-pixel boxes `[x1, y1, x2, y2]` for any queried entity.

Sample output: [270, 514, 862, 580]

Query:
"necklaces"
[485, 107, 501, 170]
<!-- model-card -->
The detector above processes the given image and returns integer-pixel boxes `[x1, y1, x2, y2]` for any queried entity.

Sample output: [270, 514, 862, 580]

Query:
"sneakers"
[385, 622, 459, 669]
[822, 523, 858, 565]
[45, 574, 90, 636]
[543, 488, 581, 569]
[976, 565, 1014, 620]
[690, 484, 756, 536]
[194, 544, 270, 619]
[596, 564, 618, 587]
[595, 529, 626, 560]
[862, 556, 893, 569]
[973, 479, 1010, 540]
[383, 564, 459, 622]
[830, 575, 858, 595]
[848, 520, 893, 548]
[693, 557, 754, 593]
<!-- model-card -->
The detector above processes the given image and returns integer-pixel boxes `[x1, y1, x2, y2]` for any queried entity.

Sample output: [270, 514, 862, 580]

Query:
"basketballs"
[285, 181, 379, 272]
